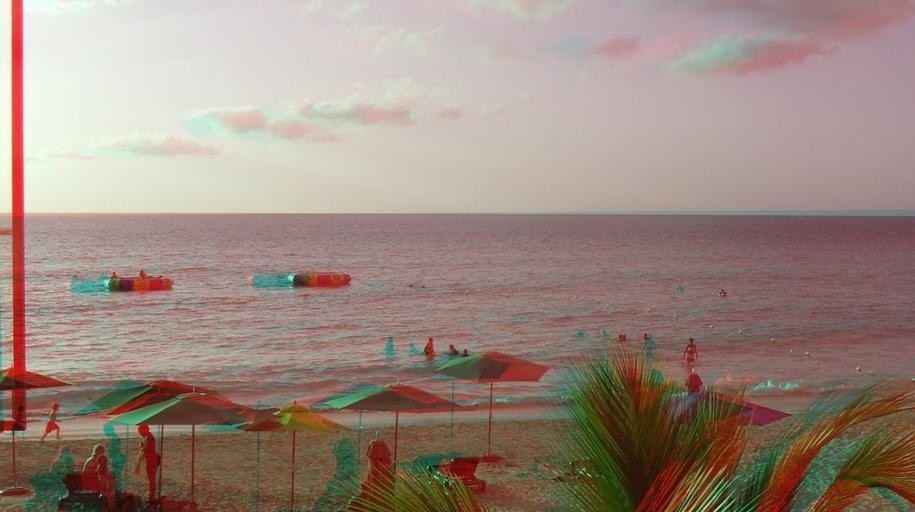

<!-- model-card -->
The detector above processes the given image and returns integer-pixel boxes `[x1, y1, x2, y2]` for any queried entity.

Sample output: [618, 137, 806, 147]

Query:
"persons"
[110, 271, 117, 279]
[719, 288, 726, 296]
[686, 368, 705, 394]
[682, 337, 700, 367]
[446, 343, 459, 357]
[140, 268, 146, 280]
[423, 336, 437, 361]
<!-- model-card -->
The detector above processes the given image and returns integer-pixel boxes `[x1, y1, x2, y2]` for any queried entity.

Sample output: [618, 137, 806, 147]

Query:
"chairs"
[58, 470, 137, 512]
[437, 456, 487, 494]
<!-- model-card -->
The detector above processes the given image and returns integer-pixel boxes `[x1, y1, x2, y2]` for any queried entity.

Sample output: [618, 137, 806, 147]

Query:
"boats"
[109, 276, 174, 291]
[289, 272, 352, 288]
